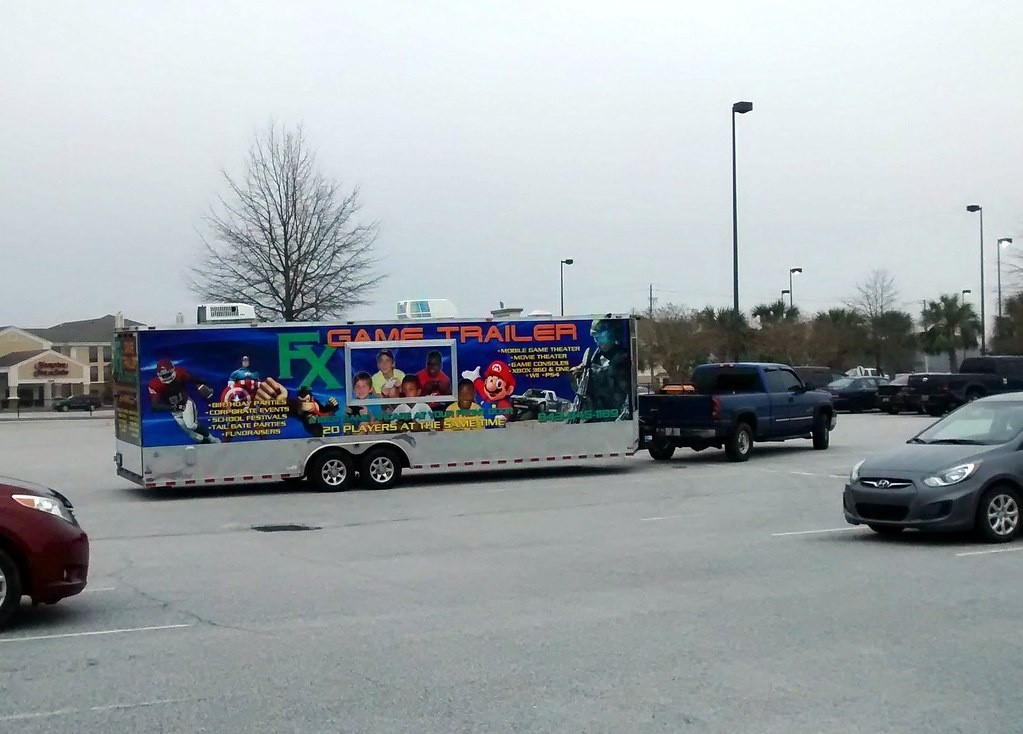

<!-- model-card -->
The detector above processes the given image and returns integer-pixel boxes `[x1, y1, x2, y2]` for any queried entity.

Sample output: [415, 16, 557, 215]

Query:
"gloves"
[174, 403, 186, 413]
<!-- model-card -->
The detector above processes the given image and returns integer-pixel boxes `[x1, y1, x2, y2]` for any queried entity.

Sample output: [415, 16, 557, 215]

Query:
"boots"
[206, 433, 221, 443]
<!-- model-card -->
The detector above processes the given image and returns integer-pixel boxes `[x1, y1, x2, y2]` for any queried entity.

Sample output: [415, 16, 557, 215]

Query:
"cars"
[877, 372, 930, 415]
[843, 390, 1023, 543]
[0, 475, 91, 633]
[52, 394, 102, 412]
[792, 365, 890, 389]
[825, 376, 890, 414]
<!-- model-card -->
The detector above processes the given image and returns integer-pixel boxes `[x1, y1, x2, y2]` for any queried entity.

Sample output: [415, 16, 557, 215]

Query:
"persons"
[566, 320, 631, 424]
[352, 348, 488, 435]
[147, 359, 222, 445]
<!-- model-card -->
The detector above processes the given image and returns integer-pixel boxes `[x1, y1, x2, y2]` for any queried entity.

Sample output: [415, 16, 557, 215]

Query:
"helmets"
[156, 360, 176, 384]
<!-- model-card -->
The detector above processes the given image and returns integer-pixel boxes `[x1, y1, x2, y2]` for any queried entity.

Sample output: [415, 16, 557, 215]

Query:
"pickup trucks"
[907, 355, 1023, 417]
[638, 362, 837, 463]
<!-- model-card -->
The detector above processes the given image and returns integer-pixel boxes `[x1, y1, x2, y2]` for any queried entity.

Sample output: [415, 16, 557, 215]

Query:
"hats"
[378, 350, 393, 359]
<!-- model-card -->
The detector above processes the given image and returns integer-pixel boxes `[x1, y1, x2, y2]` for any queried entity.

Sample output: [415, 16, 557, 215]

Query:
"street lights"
[789, 268, 803, 309]
[966, 204, 986, 356]
[780, 289, 791, 319]
[731, 100, 754, 363]
[961, 289, 972, 359]
[560, 257, 574, 316]
[997, 238, 1012, 318]
